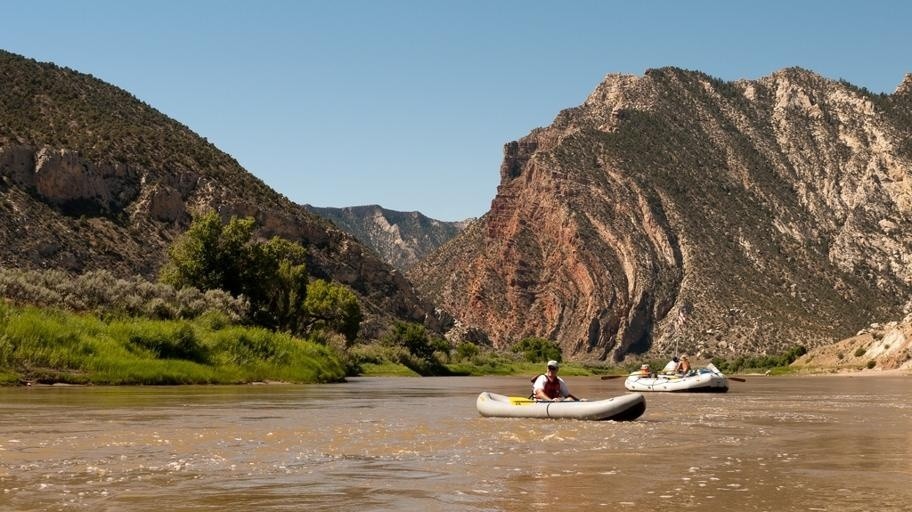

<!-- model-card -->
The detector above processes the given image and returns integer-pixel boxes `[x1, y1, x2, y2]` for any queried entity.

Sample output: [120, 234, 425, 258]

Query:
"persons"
[663, 357, 679, 374]
[641, 364, 657, 378]
[676, 355, 691, 374]
[533, 360, 589, 402]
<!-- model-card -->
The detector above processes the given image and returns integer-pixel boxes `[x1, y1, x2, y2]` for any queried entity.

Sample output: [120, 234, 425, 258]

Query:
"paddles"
[601, 374, 652, 380]
[510, 397, 579, 405]
[724, 377, 746, 382]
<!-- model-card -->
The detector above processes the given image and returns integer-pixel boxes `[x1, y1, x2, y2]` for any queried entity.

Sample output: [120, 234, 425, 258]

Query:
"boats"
[477, 390, 646, 421]
[623, 369, 729, 393]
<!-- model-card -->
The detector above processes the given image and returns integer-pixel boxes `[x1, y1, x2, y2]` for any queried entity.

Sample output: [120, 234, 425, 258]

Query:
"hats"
[548, 360, 558, 368]
